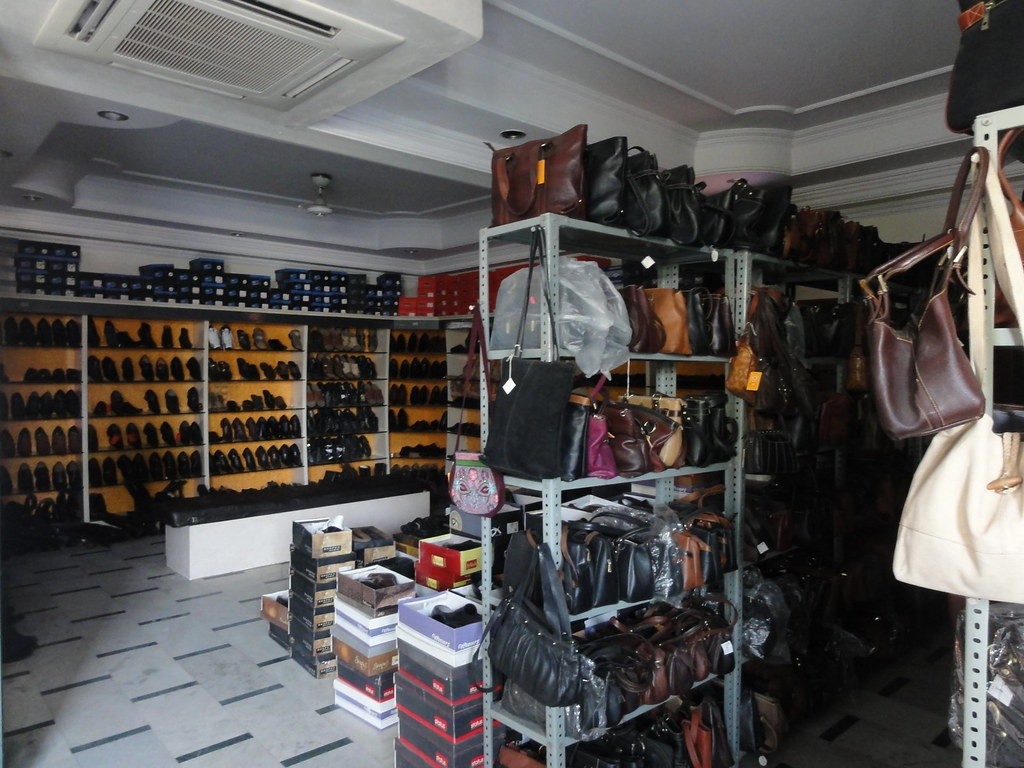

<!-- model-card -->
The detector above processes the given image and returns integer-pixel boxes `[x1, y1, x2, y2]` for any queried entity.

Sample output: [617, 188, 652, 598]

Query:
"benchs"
[166, 482, 414, 581]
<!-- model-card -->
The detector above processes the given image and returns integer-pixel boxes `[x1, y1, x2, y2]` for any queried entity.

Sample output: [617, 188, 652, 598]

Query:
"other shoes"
[0, 572, 38, 662]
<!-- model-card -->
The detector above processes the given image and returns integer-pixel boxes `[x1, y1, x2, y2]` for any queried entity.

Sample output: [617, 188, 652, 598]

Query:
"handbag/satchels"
[449, 1, 1024, 768]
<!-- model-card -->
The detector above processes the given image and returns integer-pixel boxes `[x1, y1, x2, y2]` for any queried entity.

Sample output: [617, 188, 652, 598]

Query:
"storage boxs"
[258, 513, 509, 768]
[1, 234, 610, 317]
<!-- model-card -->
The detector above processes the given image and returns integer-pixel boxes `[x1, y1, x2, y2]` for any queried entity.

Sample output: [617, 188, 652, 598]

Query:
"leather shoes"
[276, 515, 483, 638]
[0, 314, 486, 551]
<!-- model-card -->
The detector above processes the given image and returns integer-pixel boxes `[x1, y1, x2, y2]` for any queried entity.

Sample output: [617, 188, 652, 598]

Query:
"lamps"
[305, 172, 334, 218]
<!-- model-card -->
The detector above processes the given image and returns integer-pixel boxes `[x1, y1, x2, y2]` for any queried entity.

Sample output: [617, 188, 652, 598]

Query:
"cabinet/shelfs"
[1, 294, 482, 553]
[961, 103, 1024, 768]
[461, 215, 929, 768]
